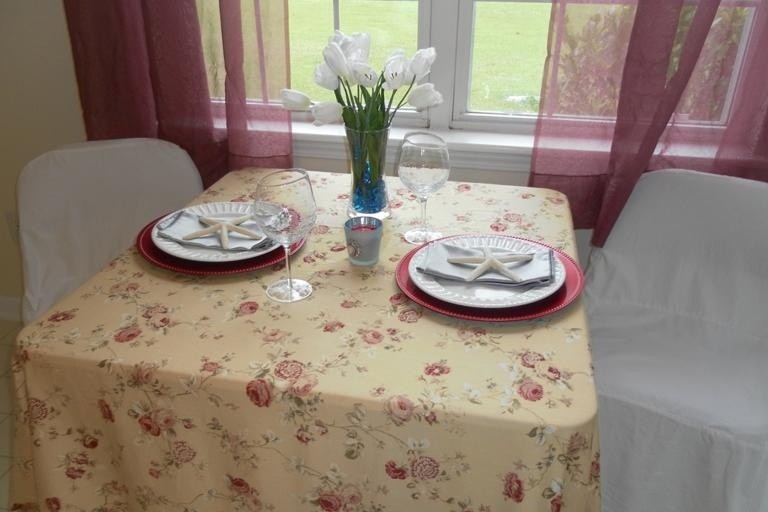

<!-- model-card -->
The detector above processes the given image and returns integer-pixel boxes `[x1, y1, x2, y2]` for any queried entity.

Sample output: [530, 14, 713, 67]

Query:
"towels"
[158, 211, 273, 253]
[418, 242, 555, 291]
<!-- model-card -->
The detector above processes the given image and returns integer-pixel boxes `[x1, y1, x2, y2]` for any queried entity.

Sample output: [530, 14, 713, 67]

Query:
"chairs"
[581, 169, 768, 512]
[16, 137, 204, 330]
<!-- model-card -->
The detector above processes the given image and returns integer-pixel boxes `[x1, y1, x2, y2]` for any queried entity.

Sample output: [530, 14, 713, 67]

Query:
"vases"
[342, 119, 395, 221]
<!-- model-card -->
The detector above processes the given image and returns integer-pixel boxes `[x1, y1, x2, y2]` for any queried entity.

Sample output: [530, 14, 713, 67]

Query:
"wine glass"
[253, 167, 314, 303]
[396, 130, 452, 246]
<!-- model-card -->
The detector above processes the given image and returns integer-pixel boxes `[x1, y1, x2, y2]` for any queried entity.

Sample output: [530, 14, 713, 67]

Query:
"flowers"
[279, 29, 445, 196]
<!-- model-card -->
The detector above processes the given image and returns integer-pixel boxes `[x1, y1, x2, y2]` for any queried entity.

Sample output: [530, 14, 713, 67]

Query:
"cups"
[344, 215, 384, 267]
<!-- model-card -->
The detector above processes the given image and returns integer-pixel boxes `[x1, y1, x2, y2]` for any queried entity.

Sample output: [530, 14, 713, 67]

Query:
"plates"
[393, 233, 586, 323]
[135, 201, 310, 277]
[150, 200, 290, 264]
[406, 234, 568, 311]
